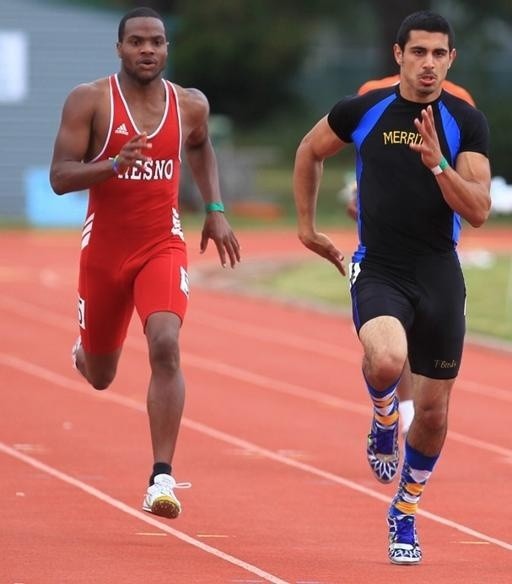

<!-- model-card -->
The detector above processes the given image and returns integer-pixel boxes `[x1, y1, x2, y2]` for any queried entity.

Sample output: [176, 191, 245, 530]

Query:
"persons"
[49, 5, 242, 520]
[346, 67, 475, 441]
[293, 7, 493, 568]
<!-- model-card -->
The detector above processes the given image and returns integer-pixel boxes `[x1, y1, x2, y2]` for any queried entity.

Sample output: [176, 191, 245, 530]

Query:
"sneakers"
[387, 515, 423, 564]
[143, 473, 181, 518]
[367, 393, 399, 483]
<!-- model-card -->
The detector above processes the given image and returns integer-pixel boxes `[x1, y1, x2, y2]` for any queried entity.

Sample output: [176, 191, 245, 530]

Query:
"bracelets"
[429, 156, 450, 176]
[205, 199, 224, 212]
[112, 153, 129, 175]
